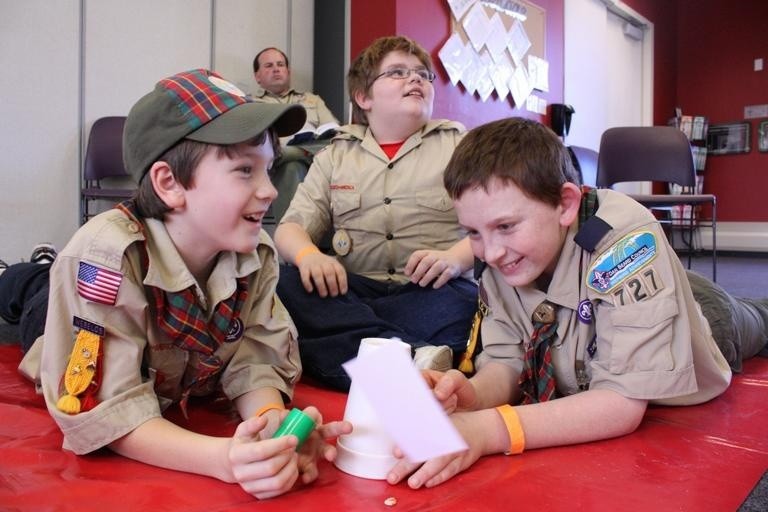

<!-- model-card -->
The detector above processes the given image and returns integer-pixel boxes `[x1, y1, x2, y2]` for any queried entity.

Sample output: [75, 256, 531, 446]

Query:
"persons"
[0, 69, 353, 500]
[274, 37, 483, 394]
[387, 117, 768, 489]
[249, 48, 340, 237]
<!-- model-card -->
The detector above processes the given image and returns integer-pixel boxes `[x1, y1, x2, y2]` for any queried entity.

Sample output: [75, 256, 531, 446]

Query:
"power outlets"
[754, 59, 762, 71]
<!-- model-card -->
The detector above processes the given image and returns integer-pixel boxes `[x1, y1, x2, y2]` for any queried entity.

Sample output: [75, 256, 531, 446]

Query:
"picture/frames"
[758, 121, 767, 150]
[705, 124, 751, 154]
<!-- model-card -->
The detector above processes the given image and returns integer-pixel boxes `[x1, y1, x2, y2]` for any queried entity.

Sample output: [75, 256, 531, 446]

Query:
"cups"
[329, 338, 429, 480]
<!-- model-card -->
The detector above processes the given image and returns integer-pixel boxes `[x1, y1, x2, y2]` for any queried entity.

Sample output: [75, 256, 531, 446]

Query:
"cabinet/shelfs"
[668, 110, 706, 257]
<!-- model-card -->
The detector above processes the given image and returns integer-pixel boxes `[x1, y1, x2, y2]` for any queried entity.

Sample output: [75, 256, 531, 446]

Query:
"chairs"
[84, 118, 133, 217]
[598, 126, 716, 285]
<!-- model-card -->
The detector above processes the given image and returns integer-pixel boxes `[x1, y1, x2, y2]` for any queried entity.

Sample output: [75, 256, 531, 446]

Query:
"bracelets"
[252, 402, 285, 417]
[295, 245, 321, 264]
[496, 404, 524, 455]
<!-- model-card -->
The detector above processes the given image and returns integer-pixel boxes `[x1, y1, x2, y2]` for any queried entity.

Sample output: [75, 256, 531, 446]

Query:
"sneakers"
[0, 256, 10, 277]
[28, 241, 59, 266]
[413, 345, 453, 375]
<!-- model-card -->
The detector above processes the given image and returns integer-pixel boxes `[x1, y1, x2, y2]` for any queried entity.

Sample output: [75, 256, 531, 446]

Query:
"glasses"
[366, 68, 436, 90]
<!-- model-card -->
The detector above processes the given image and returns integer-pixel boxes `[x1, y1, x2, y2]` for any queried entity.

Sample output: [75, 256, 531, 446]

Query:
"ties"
[148, 277, 249, 417]
[516, 322, 561, 404]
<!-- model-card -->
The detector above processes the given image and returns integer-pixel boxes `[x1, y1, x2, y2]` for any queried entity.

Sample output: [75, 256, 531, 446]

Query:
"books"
[670, 108, 708, 226]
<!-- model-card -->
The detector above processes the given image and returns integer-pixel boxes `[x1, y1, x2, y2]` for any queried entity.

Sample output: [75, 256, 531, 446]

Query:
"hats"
[120, 67, 308, 184]
